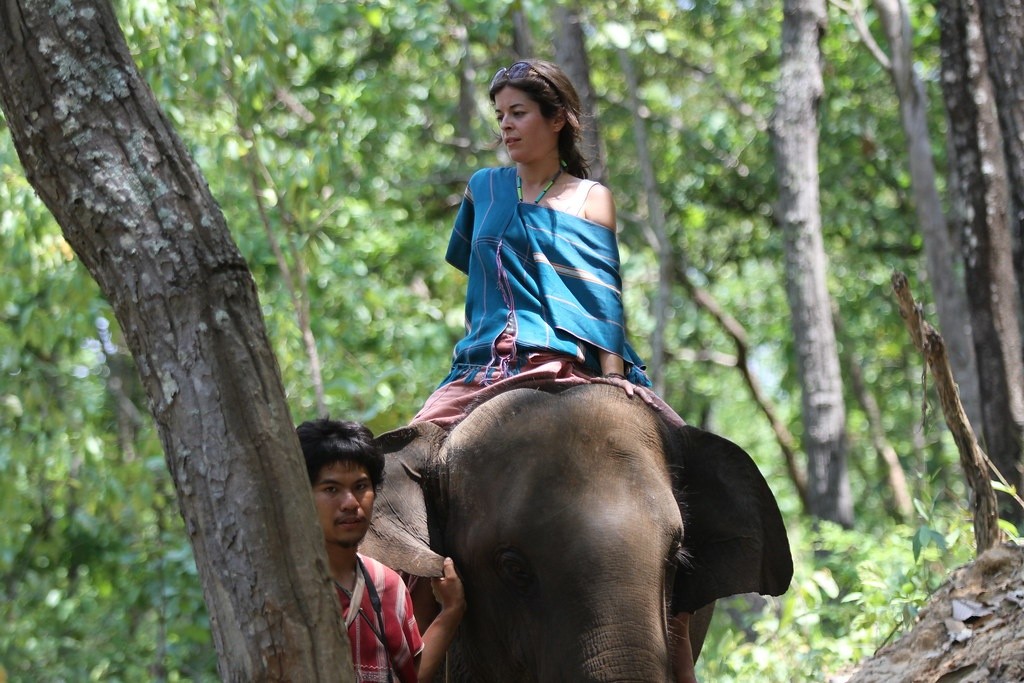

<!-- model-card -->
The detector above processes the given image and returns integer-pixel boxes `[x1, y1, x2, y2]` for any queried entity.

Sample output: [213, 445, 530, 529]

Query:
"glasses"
[488, 61, 549, 88]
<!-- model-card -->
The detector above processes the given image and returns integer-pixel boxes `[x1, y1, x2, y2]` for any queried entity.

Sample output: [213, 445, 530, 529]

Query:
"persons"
[295, 418, 463, 683]
[409, 58, 685, 427]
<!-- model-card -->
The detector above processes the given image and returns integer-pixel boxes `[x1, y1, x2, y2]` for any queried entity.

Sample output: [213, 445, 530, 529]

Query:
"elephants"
[373, 380, 794, 683]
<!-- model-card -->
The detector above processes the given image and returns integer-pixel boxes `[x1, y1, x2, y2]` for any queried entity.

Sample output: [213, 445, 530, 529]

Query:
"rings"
[633, 385, 637, 390]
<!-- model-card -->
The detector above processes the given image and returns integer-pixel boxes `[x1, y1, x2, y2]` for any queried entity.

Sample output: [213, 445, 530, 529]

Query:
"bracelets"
[603, 373, 625, 378]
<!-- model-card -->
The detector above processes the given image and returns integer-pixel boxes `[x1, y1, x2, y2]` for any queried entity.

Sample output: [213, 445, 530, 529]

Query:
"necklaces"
[516, 160, 569, 204]
